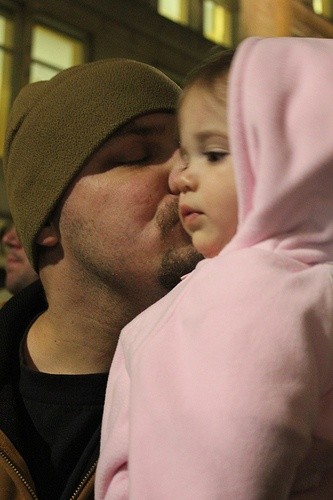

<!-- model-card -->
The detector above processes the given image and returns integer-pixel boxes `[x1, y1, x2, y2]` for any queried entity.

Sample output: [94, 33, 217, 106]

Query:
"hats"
[3, 58, 183, 274]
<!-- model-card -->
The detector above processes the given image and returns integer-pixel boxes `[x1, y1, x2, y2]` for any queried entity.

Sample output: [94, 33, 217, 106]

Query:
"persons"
[94, 34, 332, 500]
[0, 224, 39, 295]
[0, 59, 204, 500]
